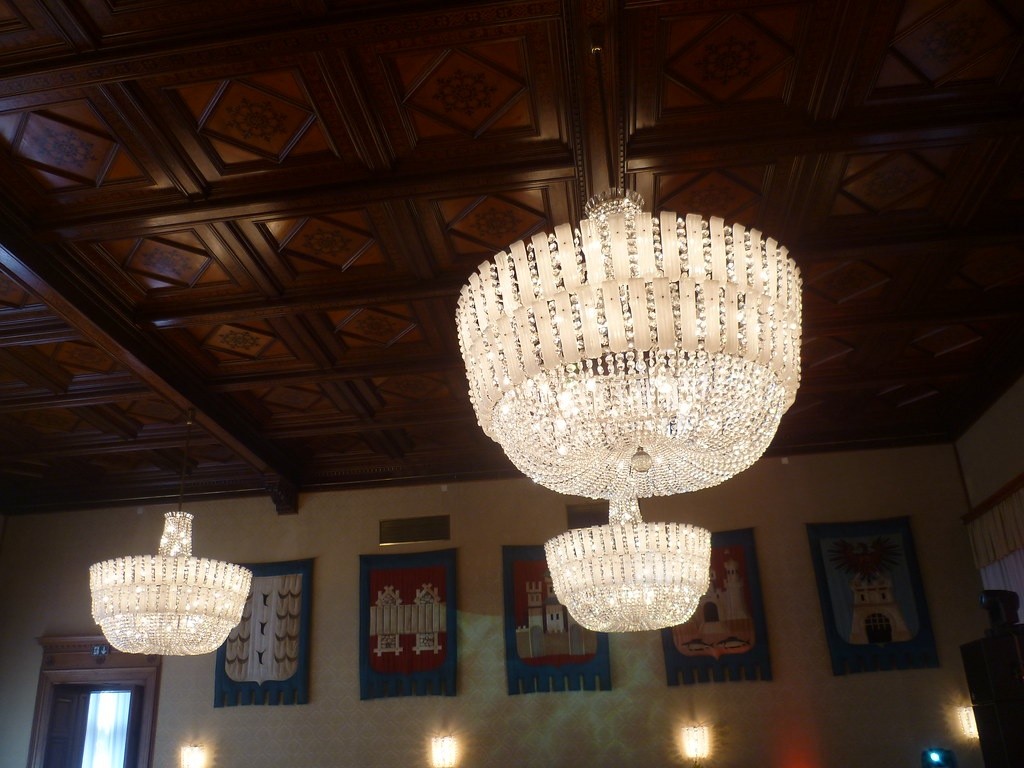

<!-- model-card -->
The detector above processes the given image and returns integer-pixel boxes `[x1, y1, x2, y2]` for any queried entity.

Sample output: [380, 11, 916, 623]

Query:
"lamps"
[682, 726, 710, 768]
[87, 407, 253, 658]
[920, 748, 957, 768]
[453, 20, 803, 636]
